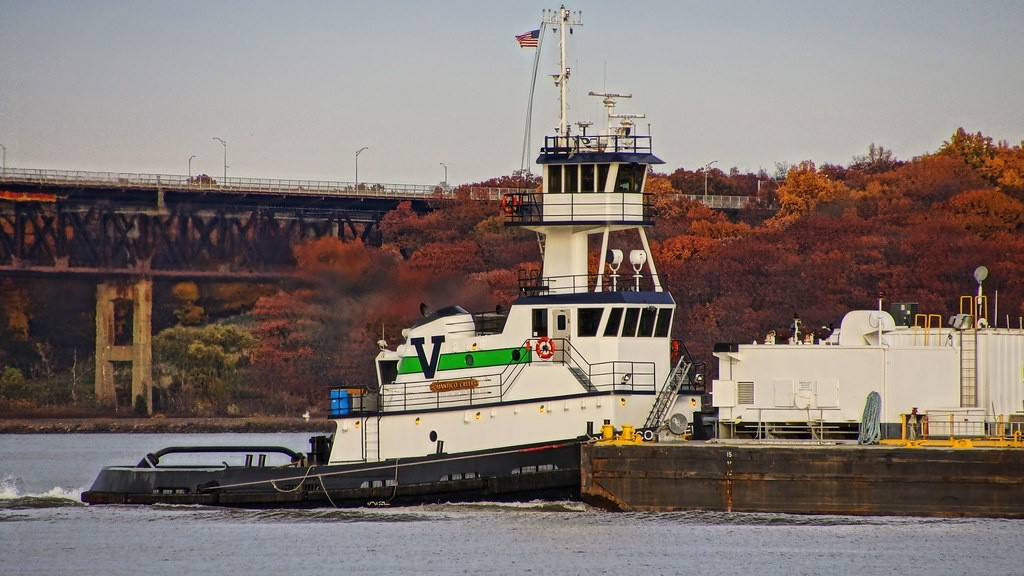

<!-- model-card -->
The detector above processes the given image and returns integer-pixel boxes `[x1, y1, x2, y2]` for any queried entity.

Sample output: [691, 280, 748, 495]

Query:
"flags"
[515, 30, 539, 48]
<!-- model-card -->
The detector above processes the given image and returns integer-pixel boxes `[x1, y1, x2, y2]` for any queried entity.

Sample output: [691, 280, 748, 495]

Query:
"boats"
[75, 3, 712, 510]
[707, 266, 1024, 438]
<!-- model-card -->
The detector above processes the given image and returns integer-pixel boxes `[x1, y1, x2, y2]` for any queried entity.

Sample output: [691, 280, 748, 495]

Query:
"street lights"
[212, 137, 227, 186]
[189, 156, 195, 176]
[356, 146, 369, 195]
[439, 162, 447, 184]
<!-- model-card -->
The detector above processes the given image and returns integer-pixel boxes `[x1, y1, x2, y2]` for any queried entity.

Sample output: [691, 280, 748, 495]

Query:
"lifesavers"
[536, 337, 556, 359]
[502, 193, 523, 214]
[671, 338, 679, 361]
